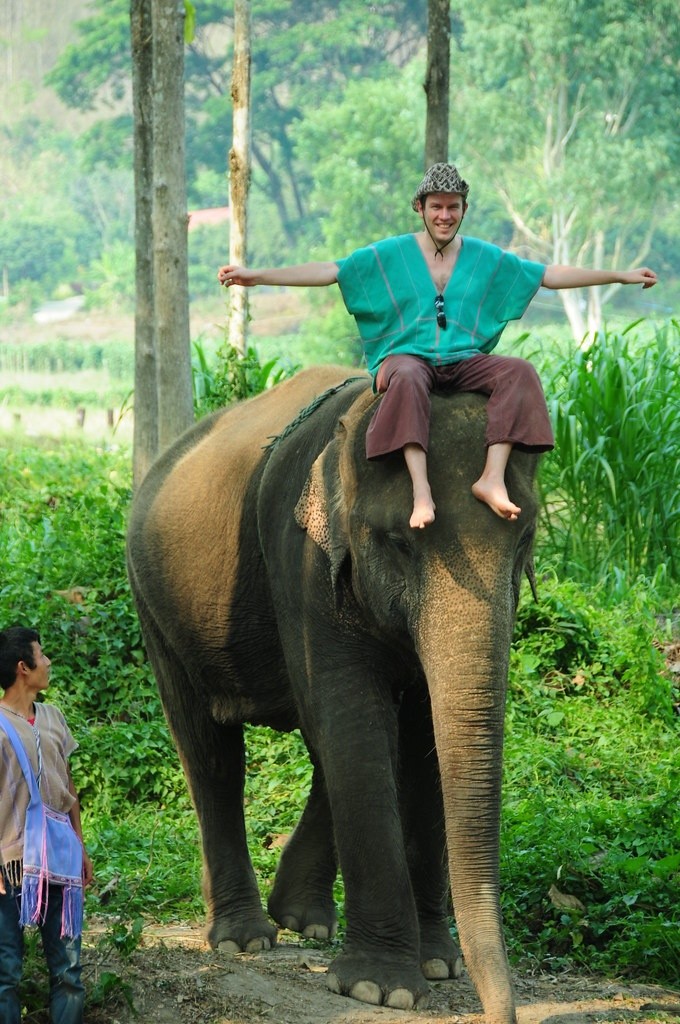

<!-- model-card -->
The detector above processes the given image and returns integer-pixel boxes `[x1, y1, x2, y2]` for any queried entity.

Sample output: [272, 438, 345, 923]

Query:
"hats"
[411, 163, 470, 260]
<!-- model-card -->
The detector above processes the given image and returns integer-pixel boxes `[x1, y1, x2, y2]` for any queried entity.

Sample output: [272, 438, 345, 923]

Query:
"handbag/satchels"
[18, 800, 83, 940]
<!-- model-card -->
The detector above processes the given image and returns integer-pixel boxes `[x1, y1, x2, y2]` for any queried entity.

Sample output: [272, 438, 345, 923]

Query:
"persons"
[0, 627, 94, 1024]
[219, 161, 658, 528]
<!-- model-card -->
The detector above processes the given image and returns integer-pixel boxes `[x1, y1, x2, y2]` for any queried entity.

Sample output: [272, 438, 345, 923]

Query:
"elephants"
[128, 364, 542, 1024]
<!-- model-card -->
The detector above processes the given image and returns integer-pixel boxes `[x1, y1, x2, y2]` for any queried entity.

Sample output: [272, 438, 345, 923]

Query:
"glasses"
[435, 293, 446, 330]
